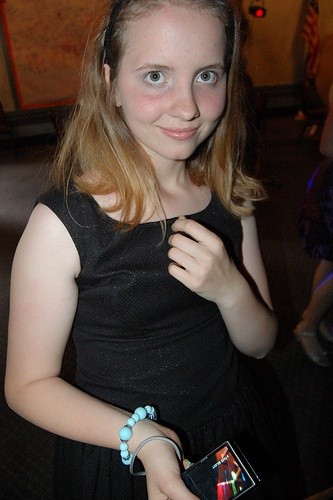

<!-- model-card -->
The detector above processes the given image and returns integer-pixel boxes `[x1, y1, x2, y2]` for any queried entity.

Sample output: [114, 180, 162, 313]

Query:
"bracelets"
[119, 404, 157, 466]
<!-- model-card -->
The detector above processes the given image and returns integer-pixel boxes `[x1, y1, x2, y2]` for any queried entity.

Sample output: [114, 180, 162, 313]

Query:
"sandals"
[294, 322, 330, 367]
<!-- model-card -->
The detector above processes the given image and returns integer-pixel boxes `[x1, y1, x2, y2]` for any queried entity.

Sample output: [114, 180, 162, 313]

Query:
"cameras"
[180, 439, 260, 500]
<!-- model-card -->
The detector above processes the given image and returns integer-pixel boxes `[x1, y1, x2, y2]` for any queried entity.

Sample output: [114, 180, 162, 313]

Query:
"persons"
[292, 0, 333, 368]
[3, 1, 320, 500]
